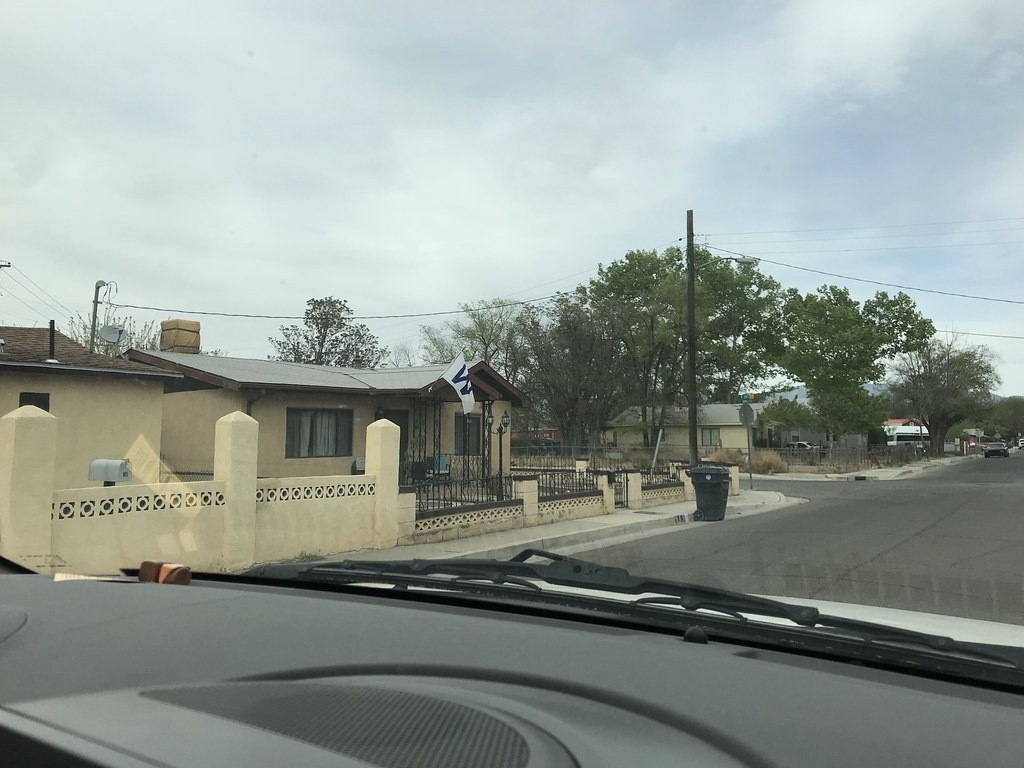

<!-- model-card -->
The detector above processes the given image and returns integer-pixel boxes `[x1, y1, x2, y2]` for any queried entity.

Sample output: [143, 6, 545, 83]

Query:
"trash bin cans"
[686, 466, 731, 522]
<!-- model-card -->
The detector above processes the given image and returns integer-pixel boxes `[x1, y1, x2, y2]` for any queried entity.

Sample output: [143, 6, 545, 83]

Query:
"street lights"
[486, 409, 510, 501]
[687, 257, 756, 466]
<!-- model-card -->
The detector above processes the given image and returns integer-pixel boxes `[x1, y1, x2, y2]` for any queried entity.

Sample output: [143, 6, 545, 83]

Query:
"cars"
[985, 443, 1009, 458]
